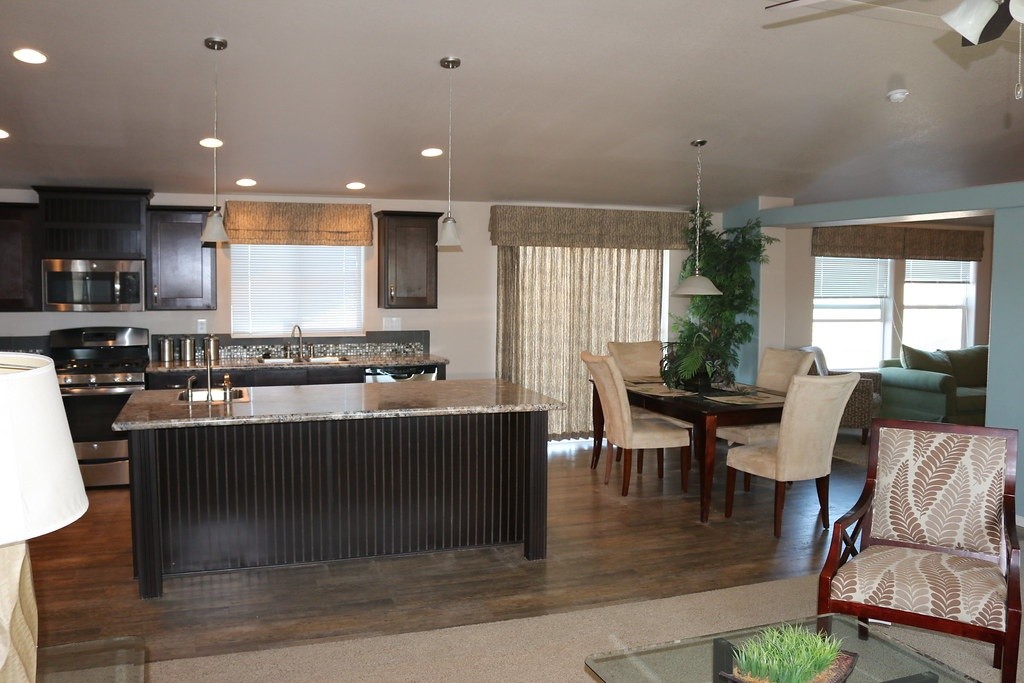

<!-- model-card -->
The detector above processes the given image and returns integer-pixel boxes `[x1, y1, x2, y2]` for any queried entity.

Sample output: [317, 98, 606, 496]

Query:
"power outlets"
[383, 317, 402, 331]
[197, 319, 207, 334]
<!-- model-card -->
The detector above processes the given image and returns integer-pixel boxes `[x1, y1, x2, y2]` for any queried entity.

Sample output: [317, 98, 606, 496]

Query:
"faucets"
[292, 325, 303, 362]
[206, 348, 212, 402]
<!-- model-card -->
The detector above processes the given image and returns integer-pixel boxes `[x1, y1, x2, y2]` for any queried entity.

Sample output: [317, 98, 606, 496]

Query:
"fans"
[761, 0, 1024, 46]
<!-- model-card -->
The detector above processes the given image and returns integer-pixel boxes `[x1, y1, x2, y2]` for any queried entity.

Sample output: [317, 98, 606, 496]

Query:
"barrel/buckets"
[180, 336, 195, 360]
[159, 336, 173, 361]
[203, 334, 219, 359]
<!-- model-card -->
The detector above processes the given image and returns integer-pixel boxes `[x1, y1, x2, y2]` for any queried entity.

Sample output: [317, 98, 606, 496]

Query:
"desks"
[588, 377, 787, 523]
[583, 612, 981, 683]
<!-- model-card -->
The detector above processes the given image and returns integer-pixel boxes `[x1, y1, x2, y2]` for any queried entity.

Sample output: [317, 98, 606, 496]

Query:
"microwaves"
[42, 259, 145, 312]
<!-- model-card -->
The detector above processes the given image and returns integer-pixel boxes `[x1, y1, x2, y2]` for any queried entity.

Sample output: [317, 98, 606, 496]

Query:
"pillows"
[901, 345, 989, 388]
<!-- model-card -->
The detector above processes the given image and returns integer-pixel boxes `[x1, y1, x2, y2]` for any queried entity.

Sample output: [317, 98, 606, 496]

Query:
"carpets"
[36, 567, 1024, 683]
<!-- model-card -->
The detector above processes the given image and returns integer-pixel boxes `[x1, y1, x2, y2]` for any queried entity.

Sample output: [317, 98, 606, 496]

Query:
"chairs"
[584, 339, 885, 535]
[815, 416, 1020, 683]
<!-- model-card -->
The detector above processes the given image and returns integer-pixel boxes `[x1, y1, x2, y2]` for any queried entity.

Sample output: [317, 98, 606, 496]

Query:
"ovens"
[60, 385, 145, 487]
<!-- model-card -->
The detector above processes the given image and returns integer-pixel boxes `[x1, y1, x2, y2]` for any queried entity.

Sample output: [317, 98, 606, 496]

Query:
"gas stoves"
[47, 326, 150, 384]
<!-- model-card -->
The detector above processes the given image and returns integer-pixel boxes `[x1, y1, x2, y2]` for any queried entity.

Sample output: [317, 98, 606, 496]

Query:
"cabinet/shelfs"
[373, 210, 443, 309]
[0, 186, 222, 312]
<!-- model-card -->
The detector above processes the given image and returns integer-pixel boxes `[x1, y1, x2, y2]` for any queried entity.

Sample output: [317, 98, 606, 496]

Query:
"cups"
[195, 346, 204, 357]
[174, 348, 180, 359]
[219, 342, 423, 359]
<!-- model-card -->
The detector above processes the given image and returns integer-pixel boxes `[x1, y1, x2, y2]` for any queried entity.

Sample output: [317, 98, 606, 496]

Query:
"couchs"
[876, 345, 989, 428]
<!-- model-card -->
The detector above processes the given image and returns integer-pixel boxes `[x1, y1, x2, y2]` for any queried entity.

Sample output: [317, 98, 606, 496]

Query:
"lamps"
[939, 0, 1024, 45]
[436, 56, 461, 246]
[670, 138, 723, 298]
[0, 350, 90, 546]
[198, 35, 230, 242]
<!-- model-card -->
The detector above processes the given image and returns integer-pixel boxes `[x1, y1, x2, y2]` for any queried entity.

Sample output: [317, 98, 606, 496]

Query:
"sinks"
[256, 357, 348, 364]
[177, 390, 244, 401]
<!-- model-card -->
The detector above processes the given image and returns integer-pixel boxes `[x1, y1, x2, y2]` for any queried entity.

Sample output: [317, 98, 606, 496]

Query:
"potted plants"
[719, 622, 858, 683]
[665, 209, 781, 393]
